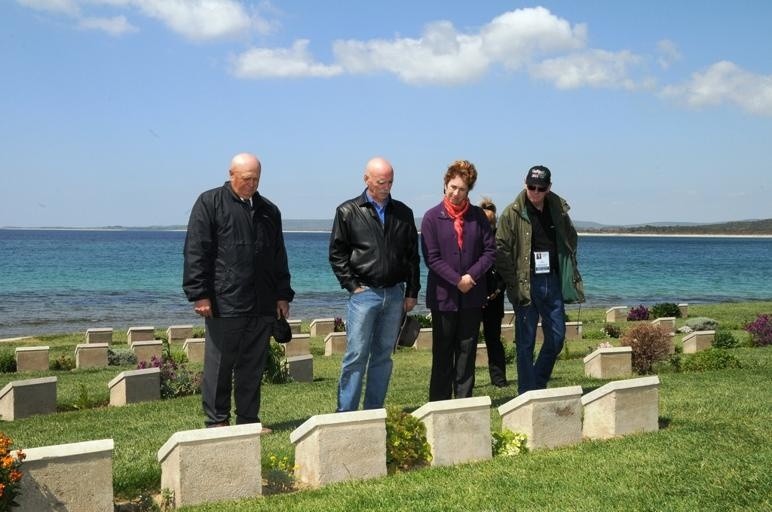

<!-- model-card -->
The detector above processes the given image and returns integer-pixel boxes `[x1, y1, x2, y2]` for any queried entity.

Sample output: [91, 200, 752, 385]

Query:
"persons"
[328, 157, 421, 413]
[496, 164, 585, 396]
[181, 153, 294, 429]
[473, 193, 510, 387]
[421, 159, 496, 403]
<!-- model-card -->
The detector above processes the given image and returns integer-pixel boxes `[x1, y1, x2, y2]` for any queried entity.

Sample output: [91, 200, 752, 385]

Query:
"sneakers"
[491, 379, 509, 387]
[207, 423, 272, 436]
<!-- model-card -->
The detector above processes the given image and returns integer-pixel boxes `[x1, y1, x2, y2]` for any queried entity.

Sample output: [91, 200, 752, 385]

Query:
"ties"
[243, 199, 251, 210]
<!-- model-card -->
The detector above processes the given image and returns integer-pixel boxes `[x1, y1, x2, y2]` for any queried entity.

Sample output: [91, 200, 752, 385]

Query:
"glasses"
[526, 184, 548, 192]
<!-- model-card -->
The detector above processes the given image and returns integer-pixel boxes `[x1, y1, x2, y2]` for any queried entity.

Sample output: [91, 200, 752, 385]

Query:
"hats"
[394, 309, 421, 354]
[273, 309, 292, 343]
[525, 166, 551, 185]
